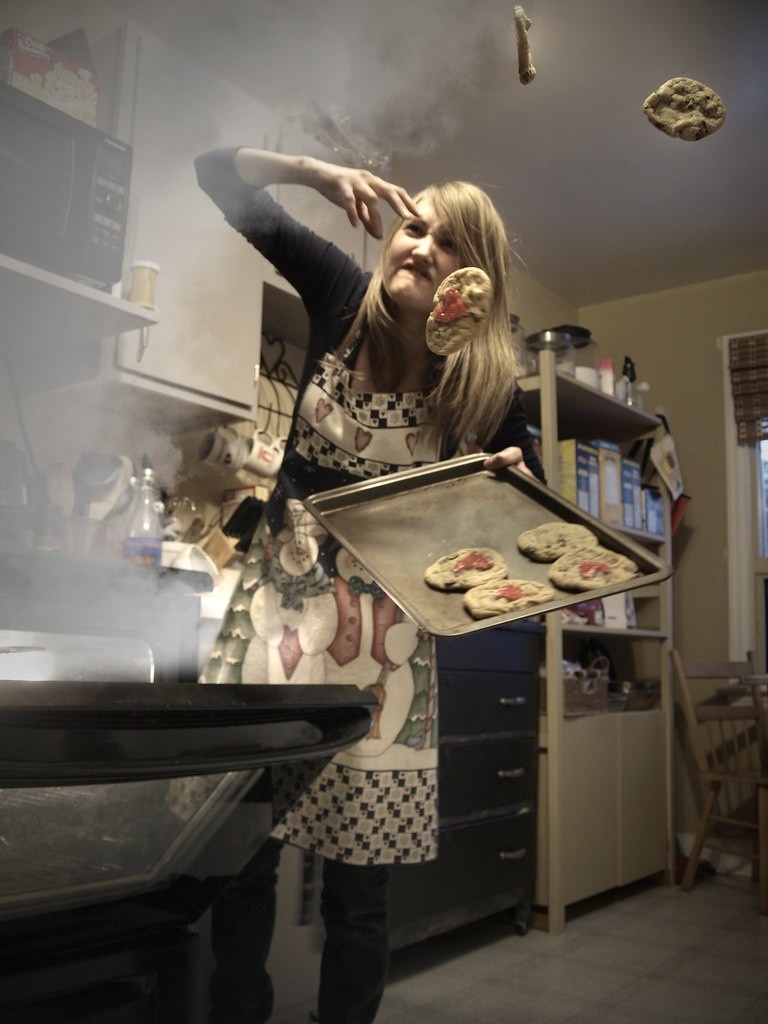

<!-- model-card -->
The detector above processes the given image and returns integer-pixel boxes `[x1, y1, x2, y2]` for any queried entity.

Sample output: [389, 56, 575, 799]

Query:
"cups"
[65, 517, 109, 560]
[195, 424, 248, 474]
[246, 428, 289, 478]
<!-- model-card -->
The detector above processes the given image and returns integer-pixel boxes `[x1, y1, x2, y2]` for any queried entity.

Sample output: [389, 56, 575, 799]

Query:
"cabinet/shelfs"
[517, 370, 672, 645]
[537, 708, 672, 933]
[382, 631, 545, 953]
[18, 21, 393, 429]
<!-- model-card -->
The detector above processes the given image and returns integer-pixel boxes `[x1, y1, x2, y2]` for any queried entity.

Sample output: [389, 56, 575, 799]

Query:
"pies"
[514, 6, 536, 85]
[425, 547, 508, 589]
[517, 523, 598, 562]
[548, 547, 638, 590]
[644, 78, 725, 140]
[425, 267, 492, 354]
[465, 581, 556, 619]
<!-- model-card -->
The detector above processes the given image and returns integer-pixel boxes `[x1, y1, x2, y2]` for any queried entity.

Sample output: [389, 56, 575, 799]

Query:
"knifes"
[222, 495, 268, 552]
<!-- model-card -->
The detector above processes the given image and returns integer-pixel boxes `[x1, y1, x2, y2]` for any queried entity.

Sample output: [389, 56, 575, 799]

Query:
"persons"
[195, 146, 549, 1024]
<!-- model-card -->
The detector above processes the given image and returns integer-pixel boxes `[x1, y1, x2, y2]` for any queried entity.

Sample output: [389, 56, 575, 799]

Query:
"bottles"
[622, 357, 637, 406]
[123, 470, 162, 565]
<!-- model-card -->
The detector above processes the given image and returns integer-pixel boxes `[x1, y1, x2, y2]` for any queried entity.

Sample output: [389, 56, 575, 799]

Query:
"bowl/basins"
[608, 679, 661, 710]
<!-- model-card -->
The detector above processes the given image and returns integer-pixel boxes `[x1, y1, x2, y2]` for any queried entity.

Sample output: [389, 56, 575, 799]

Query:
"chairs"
[671, 648, 768, 914]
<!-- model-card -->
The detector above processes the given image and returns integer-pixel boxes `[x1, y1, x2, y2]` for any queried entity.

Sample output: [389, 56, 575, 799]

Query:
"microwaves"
[0, 80, 133, 288]
